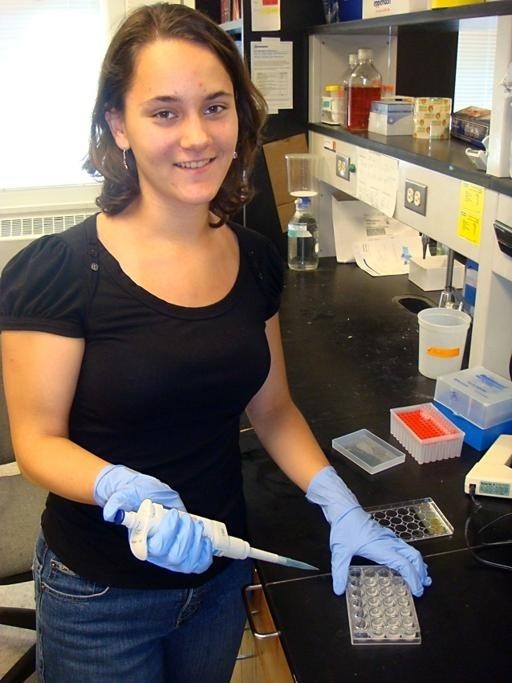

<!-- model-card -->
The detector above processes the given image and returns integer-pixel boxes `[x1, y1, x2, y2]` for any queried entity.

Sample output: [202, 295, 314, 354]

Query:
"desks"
[235, 240, 512, 683]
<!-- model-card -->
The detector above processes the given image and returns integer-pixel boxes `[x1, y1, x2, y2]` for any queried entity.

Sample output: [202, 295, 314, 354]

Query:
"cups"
[284, 151, 323, 197]
[417, 306, 472, 379]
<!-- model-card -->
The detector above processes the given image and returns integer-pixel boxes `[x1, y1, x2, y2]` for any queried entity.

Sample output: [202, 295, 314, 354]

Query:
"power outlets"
[332, 152, 351, 182]
[401, 180, 430, 215]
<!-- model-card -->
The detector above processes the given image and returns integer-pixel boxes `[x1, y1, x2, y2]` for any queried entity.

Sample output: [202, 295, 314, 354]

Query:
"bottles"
[347, 47, 382, 132]
[287, 197, 320, 270]
[338, 52, 358, 127]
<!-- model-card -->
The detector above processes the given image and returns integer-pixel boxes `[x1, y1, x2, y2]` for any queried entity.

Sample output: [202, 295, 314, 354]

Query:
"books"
[231, 0, 241, 21]
[220, 0, 231, 24]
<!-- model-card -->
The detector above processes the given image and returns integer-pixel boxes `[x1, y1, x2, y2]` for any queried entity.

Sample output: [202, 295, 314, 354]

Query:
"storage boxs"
[431, 365, 512, 452]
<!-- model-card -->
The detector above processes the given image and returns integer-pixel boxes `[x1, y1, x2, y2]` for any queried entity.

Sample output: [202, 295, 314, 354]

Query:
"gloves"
[305, 467, 431, 596]
[94, 464, 213, 574]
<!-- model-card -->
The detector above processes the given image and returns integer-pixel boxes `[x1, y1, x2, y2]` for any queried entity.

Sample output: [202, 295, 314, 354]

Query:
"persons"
[0, 2, 432, 682]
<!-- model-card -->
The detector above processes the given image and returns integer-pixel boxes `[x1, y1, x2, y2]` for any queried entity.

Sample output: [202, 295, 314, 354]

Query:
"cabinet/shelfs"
[190, 1, 306, 235]
[306, 1, 510, 377]
[232, 570, 293, 682]
